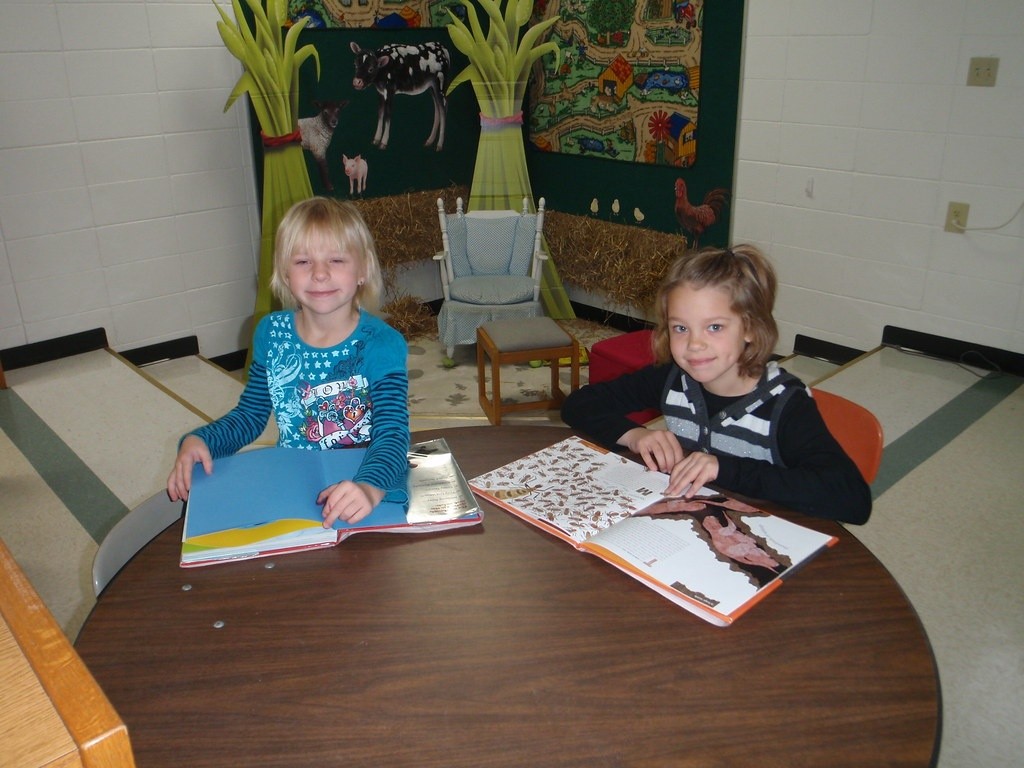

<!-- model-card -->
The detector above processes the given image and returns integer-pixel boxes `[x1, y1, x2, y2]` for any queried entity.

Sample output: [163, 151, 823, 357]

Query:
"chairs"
[432, 196, 550, 360]
[90, 488, 188, 601]
[809, 388, 884, 482]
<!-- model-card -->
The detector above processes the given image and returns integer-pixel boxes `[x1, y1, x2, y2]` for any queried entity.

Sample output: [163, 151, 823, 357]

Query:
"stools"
[587, 327, 662, 427]
[476, 316, 580, 427]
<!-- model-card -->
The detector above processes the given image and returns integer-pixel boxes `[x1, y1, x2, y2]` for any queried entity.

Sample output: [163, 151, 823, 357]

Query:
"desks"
[77, 426, 943, 768]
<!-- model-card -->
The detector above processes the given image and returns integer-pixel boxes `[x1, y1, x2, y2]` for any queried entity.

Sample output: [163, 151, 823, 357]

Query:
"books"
[467, 434, 839, 627]
[181, 438, 484, 569]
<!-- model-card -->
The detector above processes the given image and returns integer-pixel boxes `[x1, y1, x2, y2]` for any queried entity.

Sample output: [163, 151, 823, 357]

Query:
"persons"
[561, 244, 872, 525]
[168, 197, 409, 529]
